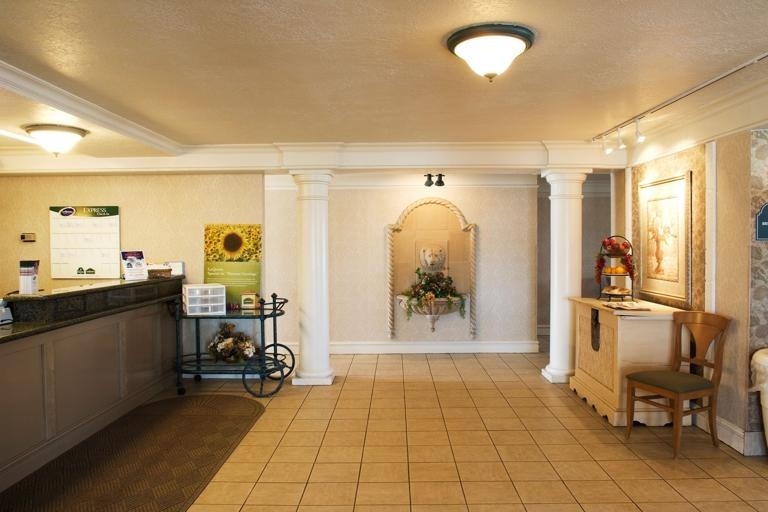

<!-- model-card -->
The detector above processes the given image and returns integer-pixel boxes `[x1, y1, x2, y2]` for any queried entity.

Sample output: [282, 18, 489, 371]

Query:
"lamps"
[19, 122, 92, 160]
[423, 173, 446, 188]
[446, 21, 536, 84]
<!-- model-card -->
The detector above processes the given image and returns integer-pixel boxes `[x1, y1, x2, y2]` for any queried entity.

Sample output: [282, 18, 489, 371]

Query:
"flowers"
[400, 266, 469, 324]
[207, 322, 256, 362]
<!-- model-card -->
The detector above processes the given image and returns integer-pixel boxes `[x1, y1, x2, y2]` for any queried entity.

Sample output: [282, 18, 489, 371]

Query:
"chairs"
[622, 309, 735, 461]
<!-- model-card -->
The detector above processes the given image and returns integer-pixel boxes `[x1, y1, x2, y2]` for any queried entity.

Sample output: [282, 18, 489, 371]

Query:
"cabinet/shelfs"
[180, 281, 226, 317]
[595, 234, 637, 304]
[565, 293, 695, 428]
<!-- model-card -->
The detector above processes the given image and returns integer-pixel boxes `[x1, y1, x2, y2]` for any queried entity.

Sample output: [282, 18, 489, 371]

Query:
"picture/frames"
[634, 167, 695, 313]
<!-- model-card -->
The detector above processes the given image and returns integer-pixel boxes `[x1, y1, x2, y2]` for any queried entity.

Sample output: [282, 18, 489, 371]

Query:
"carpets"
[0, 392, 266, 511]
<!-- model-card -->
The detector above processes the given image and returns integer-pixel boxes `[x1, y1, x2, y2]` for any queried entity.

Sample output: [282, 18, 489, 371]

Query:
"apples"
[603, 238, 630, 251]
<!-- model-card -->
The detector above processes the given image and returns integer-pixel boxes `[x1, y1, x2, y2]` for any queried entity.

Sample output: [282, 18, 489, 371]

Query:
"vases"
[220, 347, 242, 365]
